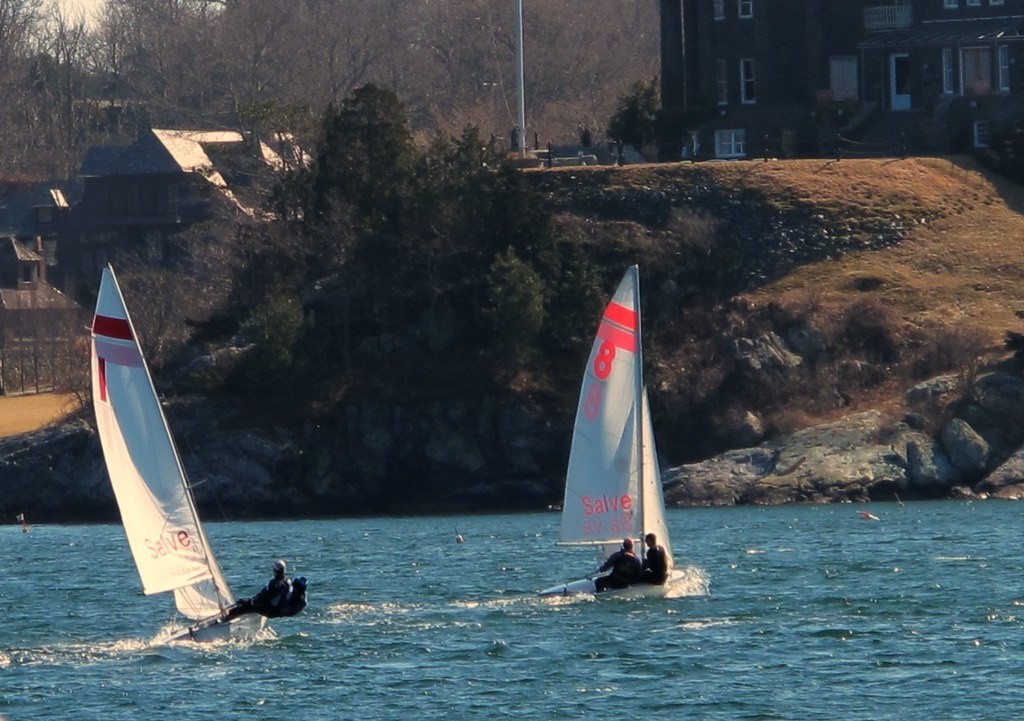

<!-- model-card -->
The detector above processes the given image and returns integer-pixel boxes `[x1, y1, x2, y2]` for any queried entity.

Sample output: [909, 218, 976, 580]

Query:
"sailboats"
[92, 262, 267, 642]
[538, 265, 686, 596]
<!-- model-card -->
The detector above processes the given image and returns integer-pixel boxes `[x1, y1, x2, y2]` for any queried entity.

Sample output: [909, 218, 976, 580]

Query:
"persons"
[594, 538, 642, 593]
[640, 533, 668, 585]
[216, 559, 308, 624]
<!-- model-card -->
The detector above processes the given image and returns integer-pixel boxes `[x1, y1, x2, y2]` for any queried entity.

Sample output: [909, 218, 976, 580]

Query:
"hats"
[624, 538, 633, 545]
[272, 560, 287, 571]
